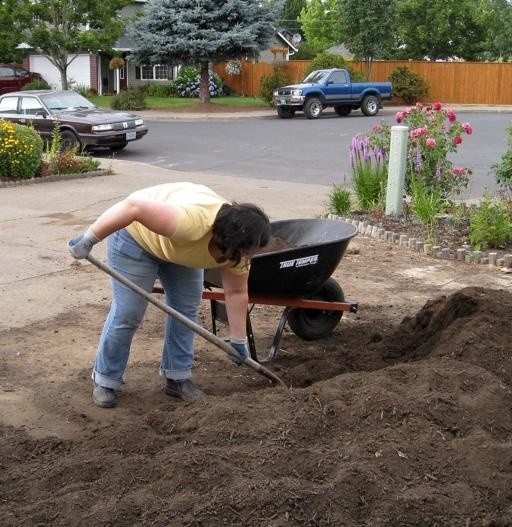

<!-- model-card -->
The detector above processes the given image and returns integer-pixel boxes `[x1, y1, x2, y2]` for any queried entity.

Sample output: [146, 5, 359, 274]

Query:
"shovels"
[84, 255, 288, 389]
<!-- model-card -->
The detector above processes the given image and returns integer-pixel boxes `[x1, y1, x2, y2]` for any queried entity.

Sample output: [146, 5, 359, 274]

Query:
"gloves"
[67, 224, 102, 260]
[230, 336, 251, 366]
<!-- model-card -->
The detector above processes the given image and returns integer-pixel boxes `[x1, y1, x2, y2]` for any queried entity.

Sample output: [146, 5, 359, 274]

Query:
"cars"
[0, 63, 149, 158]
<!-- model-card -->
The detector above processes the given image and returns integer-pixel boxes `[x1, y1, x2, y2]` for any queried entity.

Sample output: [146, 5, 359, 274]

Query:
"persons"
[67, 182, 271, 408]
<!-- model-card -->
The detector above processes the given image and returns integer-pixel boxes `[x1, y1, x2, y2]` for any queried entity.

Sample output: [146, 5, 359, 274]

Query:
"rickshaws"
[152, 217, 359, 366]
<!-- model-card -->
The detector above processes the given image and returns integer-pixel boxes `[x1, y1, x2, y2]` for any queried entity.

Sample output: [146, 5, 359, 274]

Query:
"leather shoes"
[92, 384, 116, 408]
[164, 378, 203, 401]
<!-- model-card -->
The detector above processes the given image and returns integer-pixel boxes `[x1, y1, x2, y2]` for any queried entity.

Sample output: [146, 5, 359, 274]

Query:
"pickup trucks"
[272, 67, 394, 121]
